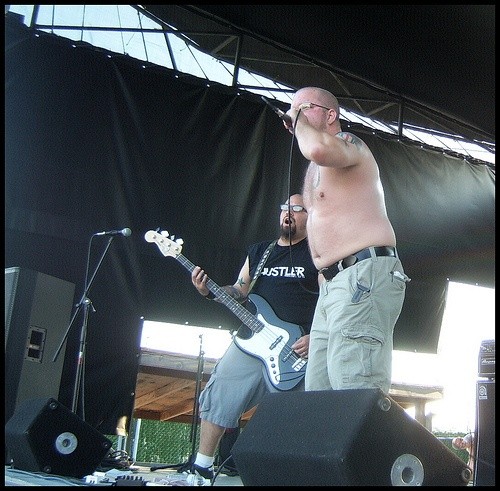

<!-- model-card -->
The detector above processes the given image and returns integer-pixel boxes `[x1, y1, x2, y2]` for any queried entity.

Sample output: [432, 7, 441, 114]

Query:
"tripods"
[149, 352, 206, 475]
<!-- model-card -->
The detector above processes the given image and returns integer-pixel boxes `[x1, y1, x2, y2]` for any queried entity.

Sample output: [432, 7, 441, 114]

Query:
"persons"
[282, 86, 410, 395]
[452, 433, 475, 480]
[176, 193, 320, 487]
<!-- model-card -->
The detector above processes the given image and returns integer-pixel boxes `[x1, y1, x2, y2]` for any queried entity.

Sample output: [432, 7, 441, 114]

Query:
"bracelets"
[205, 290, 216, 300]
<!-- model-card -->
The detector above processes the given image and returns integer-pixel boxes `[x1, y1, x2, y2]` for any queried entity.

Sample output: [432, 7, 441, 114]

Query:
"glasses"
[280, 204, 307, 214]
[294, 102, 331, 113]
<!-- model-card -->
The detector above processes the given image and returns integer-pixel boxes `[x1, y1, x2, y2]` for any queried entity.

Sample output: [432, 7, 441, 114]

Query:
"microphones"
[274, 109, 295, 134]
[95, 227, 131, 236]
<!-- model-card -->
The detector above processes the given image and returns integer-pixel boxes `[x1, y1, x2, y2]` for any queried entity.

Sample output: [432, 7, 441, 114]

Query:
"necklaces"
[310, 163, 321, 191]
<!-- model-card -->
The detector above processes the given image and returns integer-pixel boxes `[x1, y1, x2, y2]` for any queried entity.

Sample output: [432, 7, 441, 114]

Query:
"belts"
[322, 245, 397, 282]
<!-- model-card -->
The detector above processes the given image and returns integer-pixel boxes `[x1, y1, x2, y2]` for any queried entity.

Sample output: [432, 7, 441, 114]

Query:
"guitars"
[144, 227, 308, 391]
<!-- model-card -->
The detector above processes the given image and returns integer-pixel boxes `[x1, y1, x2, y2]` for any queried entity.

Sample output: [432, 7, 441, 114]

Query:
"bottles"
[166, 473, 210, 486]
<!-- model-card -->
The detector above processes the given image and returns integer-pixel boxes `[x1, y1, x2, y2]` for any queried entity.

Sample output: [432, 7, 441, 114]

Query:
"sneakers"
[181, 464, 215, 483]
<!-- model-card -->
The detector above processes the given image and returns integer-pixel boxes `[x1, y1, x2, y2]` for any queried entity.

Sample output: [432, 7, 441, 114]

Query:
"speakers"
[3, 266, 77, 458]
[5, 398, 112, 477]
[472, 382, 496, 486]
[231, 388, 473, 487]
